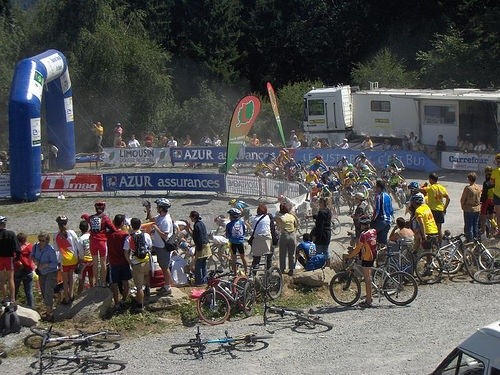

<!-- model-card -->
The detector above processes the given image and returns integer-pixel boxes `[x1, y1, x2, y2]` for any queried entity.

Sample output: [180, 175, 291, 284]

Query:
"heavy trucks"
[303, 80, 500, 153]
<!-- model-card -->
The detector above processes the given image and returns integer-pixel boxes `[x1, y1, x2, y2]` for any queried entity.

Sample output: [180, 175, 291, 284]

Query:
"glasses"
[38, 239, 45, 242]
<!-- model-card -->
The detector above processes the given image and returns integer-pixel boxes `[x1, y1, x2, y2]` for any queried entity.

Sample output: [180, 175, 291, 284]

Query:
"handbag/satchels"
[248, 235, 254, 246]
[165, 233, 179, 251]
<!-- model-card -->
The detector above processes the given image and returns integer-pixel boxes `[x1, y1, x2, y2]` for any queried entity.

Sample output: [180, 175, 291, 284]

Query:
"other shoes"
[359, 301, 372, 308]
[60, 282, 172, 314]
[41, 312, 54, 322]
[287, 269, 294, 276]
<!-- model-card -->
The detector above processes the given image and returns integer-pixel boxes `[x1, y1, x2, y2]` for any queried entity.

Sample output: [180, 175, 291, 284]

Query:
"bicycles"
[330, 229, 500, 306]
[30, 324, 125, 353]
[170, 326, 274, 360]
[263, 291, 334, 330]
[33, 350, 128, 374]
[197, 251, 283, 325]
[257, 165, 407, 236]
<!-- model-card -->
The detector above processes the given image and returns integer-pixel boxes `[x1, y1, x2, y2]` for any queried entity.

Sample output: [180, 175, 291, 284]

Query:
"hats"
[116, 123, 121, 125]
[290, 130, 296, 133]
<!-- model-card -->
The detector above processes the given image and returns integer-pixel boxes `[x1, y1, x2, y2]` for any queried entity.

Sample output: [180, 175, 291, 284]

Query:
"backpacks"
[230, 221, 244, 238]
[130, 232, 148, 259]
[0, 302, 21, 337]
[89, 214, 106, 233]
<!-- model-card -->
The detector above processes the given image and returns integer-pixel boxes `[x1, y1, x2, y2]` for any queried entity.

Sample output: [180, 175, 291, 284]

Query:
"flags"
[227, 95, 261, 173]
[267, 82, 286, 144]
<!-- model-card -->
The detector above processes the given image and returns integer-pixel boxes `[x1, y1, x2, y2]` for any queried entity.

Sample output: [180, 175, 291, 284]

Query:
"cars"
[428, 320, 500, 375]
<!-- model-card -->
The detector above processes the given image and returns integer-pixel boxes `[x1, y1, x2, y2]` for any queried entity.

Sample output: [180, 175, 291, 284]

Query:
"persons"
[128, 135, 140, 147]
[41, 143, 58, 172]
[249, 130, 500, 308]
[93, 122, 104, 152]
[145, 133, 222, 168]
[113, 123, 123, 148]
[0, 197, 253, 323]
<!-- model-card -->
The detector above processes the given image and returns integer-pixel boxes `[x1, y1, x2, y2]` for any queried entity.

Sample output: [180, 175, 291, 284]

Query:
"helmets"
[94, 201, 107, 209]
[0, 215, 8, 224]
[258, 146, 500, 224]
[227, 208, 241, 215]
[56, 215, 68, 224]
[232, 201, 245, 209]
[154, 198, 171, 209]
